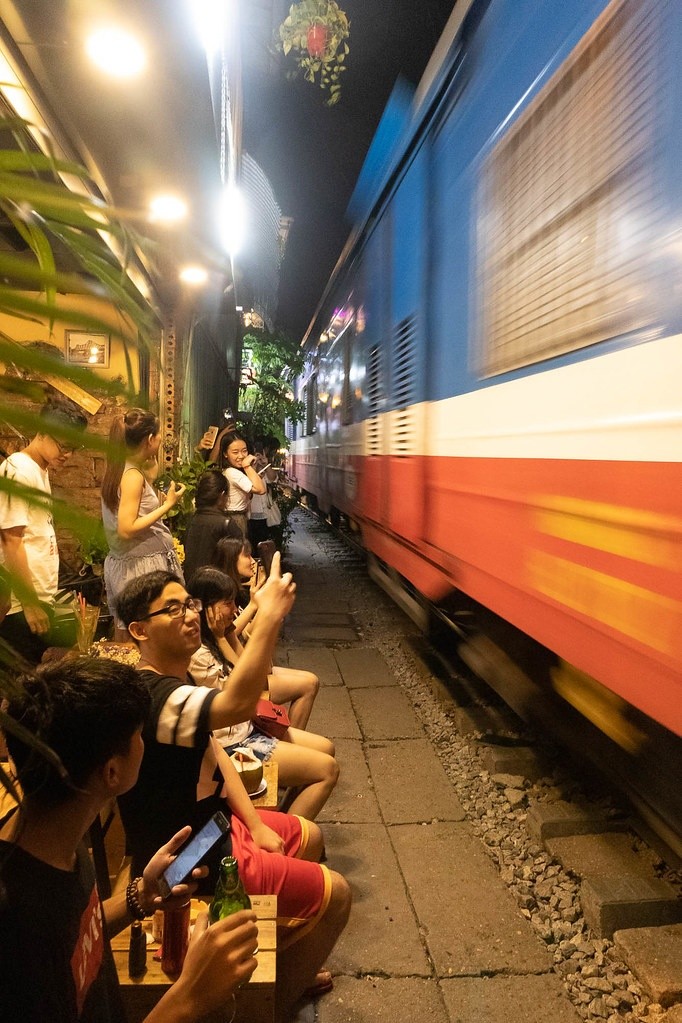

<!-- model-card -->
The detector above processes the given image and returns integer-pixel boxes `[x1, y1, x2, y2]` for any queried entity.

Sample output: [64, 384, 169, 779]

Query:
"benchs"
[108, 756, 281, 1023]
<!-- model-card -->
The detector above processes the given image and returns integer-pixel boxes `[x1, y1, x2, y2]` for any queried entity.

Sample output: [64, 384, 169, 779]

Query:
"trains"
[279, 0, 682, 767]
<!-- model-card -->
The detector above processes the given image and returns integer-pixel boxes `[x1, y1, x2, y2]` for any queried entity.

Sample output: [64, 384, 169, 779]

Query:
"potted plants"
[263, 0, 351, 108]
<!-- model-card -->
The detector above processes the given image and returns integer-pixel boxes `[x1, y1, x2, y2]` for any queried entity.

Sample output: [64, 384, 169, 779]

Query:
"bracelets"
[126, 881, 146, 921]
[130, 877, 156, 917]
[243, 465, 251, 470]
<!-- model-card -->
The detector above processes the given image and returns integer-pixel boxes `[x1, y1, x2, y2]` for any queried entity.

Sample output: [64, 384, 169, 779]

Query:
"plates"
[248, 779, 268, 796]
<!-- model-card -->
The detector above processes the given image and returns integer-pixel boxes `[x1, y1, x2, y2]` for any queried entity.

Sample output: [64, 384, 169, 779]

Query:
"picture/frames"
[63, 328, 111, 369]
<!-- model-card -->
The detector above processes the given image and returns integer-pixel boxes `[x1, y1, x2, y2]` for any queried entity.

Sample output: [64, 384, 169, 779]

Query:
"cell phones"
[157, 811, 230, 902]
[257, 463, 271, 474]
[150, 472, 177, 496]
[257, 541, 276, 578]
[205, 426, 220, 449]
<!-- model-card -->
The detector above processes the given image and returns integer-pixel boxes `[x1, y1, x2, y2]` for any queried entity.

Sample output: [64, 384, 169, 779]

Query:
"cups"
[154, 909, 162, 943]
[129, 919, 147, 978]
[162, 899, 191, 973]
[76, 608, 100, 652]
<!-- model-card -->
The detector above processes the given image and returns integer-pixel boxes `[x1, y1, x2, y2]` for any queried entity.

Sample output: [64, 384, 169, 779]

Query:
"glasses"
[50, 435, 84, 454]
[137, 599, 203, 619]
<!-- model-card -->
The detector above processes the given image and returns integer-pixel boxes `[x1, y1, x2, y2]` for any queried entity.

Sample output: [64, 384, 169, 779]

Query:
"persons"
[0, 657, 260, 1023]
[104, 408, 187, 646]
[115, 422, 352, 1022]
[0, 396, 88, 762]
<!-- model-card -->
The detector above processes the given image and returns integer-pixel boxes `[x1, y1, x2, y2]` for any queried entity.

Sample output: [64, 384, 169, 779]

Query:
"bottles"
[207, 855, 252, 926]
